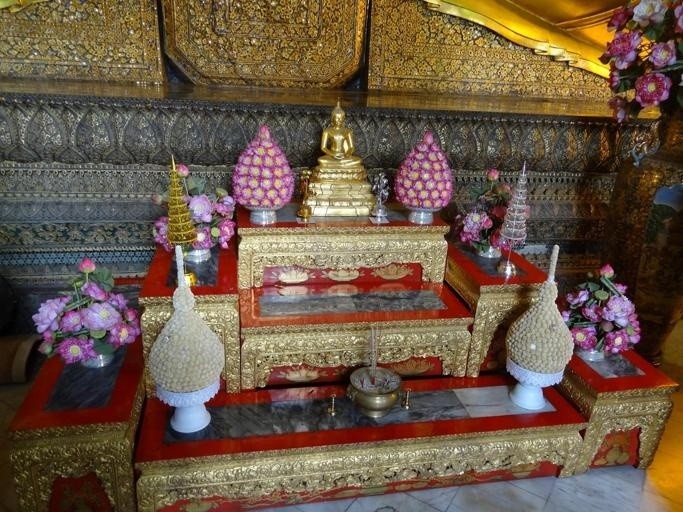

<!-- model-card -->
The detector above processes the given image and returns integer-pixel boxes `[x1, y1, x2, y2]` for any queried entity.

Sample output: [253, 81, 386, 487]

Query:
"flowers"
[459, 167, 521, 244]
[149, 163, 237, 251]
[597, 0, 682, 120]
[232, 122, 295, 209]
[392, 130, 454, 207]
